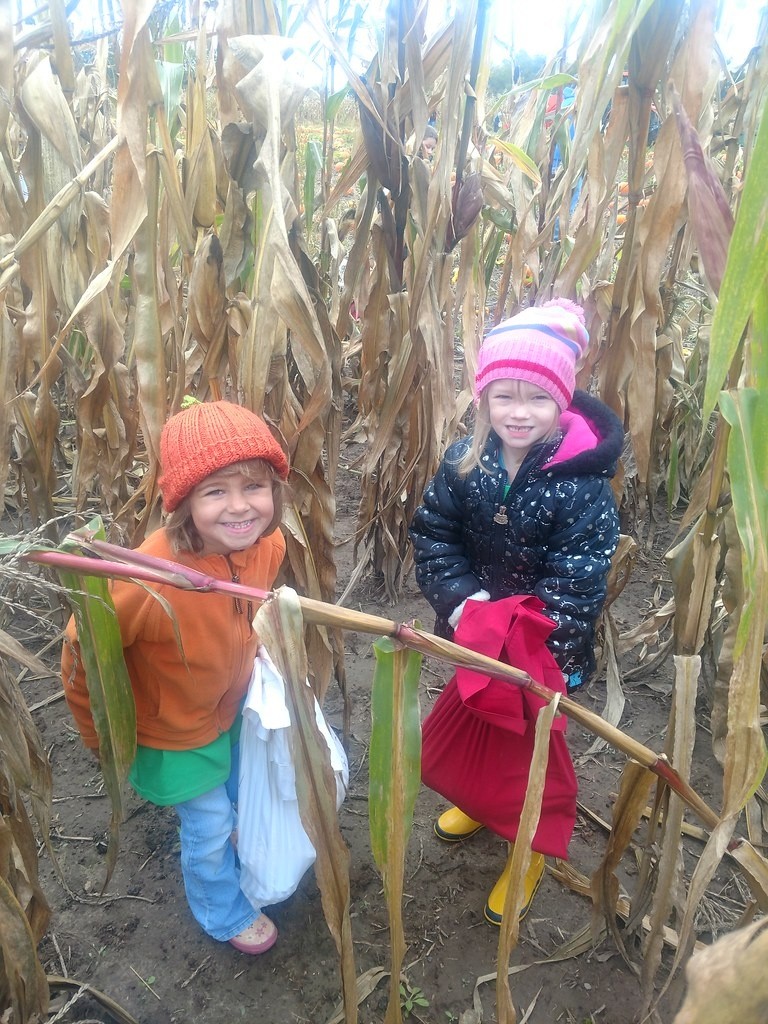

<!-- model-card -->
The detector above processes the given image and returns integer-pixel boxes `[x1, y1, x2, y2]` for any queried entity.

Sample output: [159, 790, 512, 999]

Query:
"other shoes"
[228, 912, 279, 955]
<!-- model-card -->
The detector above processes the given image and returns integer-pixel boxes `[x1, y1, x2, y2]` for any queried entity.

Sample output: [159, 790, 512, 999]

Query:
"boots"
[433, 805, 484, 842]
[484, 842, 546, 927]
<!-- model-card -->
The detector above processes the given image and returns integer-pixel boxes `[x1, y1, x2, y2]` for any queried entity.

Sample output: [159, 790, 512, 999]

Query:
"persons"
[57, 394, 292, 950]
[407, 299, 624, 924]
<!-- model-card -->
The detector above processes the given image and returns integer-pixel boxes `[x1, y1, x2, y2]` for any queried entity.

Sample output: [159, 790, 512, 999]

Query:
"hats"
[156, 394, 289, 513]
[474, 298, 591, 413]
